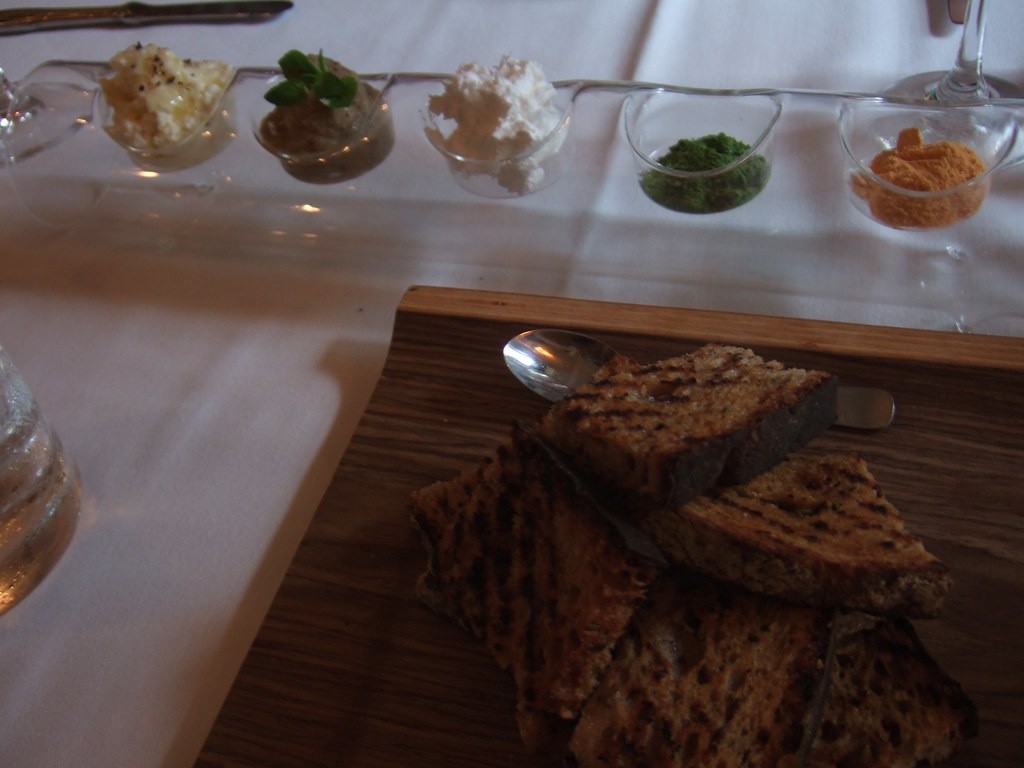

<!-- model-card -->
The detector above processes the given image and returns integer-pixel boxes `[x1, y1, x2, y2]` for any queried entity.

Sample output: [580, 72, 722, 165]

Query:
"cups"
[0, 341, 83, 613]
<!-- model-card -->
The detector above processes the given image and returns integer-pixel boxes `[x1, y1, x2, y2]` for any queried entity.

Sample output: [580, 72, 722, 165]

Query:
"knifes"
[0, 0, 293, 29]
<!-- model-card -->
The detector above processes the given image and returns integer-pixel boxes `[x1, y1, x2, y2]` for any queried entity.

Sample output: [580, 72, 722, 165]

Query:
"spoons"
[502, 328, 896, 430]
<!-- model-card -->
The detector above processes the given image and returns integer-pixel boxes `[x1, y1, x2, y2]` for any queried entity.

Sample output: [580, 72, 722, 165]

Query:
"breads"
[403, 342, 981, 768]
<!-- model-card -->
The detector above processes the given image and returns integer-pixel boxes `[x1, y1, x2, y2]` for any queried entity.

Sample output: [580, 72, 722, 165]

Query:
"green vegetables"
[261, 47, 357, 109]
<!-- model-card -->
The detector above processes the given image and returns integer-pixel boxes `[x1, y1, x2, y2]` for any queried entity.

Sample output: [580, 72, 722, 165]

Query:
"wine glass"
[884, 0, 1024, 173]
